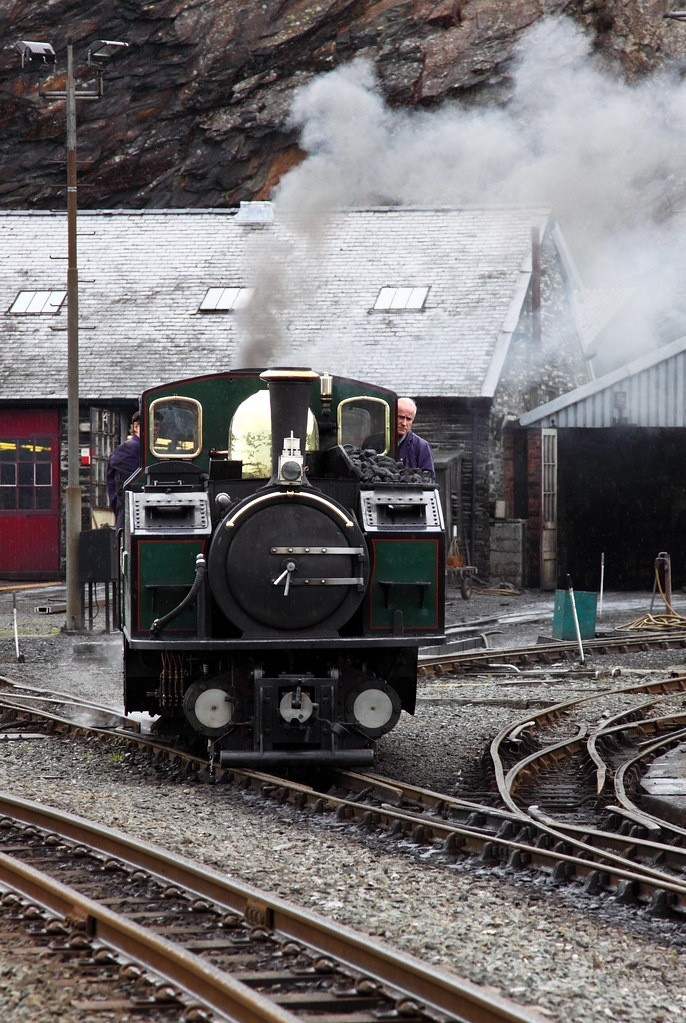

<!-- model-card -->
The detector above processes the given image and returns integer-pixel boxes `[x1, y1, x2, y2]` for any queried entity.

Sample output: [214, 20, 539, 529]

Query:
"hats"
[132, 410, 163, 422]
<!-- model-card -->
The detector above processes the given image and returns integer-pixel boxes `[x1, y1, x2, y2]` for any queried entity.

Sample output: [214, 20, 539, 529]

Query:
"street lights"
[5, 33, 128, 633]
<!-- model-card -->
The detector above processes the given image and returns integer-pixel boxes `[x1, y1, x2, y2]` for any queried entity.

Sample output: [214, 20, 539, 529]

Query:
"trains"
[111, 366, 449, 772]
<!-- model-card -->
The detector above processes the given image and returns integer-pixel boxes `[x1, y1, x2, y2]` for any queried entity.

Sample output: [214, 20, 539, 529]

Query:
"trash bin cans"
[554, 588, 598, 640]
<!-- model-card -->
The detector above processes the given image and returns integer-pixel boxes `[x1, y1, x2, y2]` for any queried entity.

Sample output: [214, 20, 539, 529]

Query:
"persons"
[106, 409, 164, 530]
[362, 398, 436, 481]
